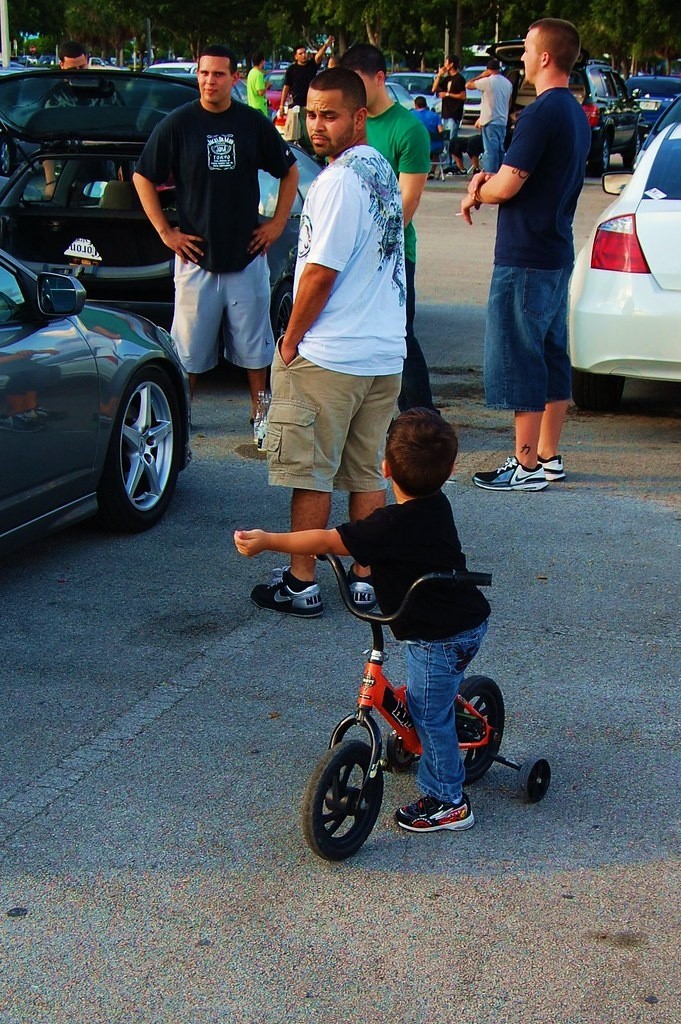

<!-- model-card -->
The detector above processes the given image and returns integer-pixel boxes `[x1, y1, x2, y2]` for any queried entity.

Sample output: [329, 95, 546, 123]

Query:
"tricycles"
[300, 552, 553, 862]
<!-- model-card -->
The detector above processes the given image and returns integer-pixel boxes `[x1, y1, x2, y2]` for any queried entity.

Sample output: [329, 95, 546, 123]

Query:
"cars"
[271, 83, 414, 145]
[238, 59, 291, 71]
[568, 122, 681, 414]
[383, 69, 440, 113]
[624, 75, 681, 173]
[1, 49, 186, 69]
[1, 68, 326, 379]
[263, 69, 291, 129]
[1, 248, 192, 560]
[433, 65, 510, 121]
[128, 63, 249, 107]
[0, 126, 41, 178]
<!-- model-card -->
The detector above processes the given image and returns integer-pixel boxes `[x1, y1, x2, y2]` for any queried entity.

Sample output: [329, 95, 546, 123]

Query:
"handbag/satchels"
[283, 106, 300, 142]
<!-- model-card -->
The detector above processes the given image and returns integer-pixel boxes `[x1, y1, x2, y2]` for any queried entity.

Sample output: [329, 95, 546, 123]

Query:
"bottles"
[264, 392, 272, 401]
[253, 391, 265, 444]
[258, 399, 269, 452]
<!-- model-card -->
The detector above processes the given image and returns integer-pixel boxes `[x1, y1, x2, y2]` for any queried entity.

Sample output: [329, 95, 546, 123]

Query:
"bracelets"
[446, 92, 450, 96]
[279, 105, 284, 108]
[45, 181, 57, 186]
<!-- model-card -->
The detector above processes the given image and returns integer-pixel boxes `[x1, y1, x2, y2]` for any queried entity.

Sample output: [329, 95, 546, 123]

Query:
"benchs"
[516, 83, 584, 106]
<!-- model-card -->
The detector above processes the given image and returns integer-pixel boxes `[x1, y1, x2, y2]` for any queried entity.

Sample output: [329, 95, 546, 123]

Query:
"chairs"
[430, 130, 450, 182]
[99, 181, 142, 209]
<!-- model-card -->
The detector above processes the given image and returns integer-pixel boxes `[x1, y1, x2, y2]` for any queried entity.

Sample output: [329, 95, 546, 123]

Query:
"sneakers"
[473, 456, 548, 491]
[448, 168, 467, 176]
[348, 562, 378, 612]
[473, 168, 481, 176]
[395, 795, 476, 831]
[537, 454, 566, 481]
[252, 566, 323, 617]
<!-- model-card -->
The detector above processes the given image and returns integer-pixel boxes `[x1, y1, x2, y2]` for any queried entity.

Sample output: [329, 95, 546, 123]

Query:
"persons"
[40, 41, 136, 200]
[249, 65, 408, 620]
[431, 55, 467, 174]
[446, 59, 525, 177]
[459, 17, 593, 492]
[233, 405, 494, 834]
[279, 35, 335, 160]
[246, 51, 273, 118]
[131, 44, 300, 450]
[408, 96, 444, 180]
[327, 42, 443, 427]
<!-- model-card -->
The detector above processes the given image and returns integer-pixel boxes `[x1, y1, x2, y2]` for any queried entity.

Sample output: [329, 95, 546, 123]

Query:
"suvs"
[487, 38, 647, 178]
